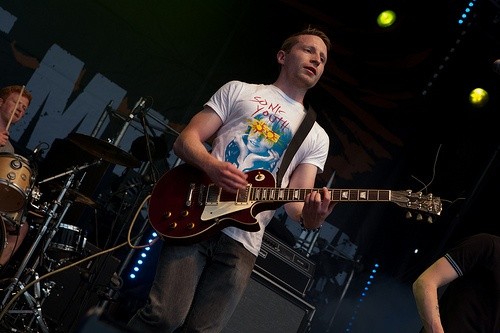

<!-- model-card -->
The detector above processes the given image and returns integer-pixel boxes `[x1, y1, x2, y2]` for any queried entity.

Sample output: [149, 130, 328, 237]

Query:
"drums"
[0, 206, 25, 235]
[0, 151, 35, 213]
[28, 218, 87, 255]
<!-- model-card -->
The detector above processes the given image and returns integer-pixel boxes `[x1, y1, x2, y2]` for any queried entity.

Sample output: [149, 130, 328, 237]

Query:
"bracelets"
[298, 213, 323, 231]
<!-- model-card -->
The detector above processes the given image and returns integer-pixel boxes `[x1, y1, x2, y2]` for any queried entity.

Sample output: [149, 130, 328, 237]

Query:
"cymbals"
[68, 132, 141, 168]
[49, 182, 95, 205]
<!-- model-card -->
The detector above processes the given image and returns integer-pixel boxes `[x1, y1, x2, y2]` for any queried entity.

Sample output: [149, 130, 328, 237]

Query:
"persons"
[0, 86, 32, 269]
[412, 233, 500, 333]
[129, 28, 339, 333]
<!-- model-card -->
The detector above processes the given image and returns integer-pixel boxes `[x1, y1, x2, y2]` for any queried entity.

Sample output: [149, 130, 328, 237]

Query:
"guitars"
[147, 162, 443, 245]
